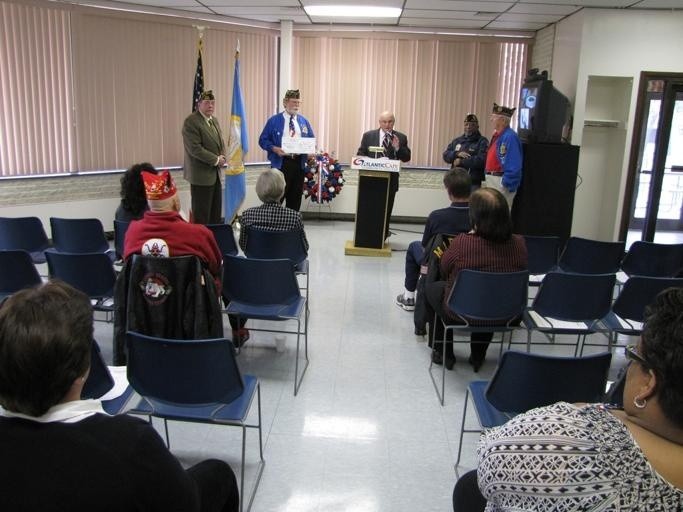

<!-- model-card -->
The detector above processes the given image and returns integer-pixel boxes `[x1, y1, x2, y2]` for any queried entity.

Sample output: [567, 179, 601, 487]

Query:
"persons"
[181, 90, 226, 227]
[452, 288, 683, 511]
[442, 115, 487, 196]
[356, 111, 411, 238]
[238, 169, 309, 271]
[122, 172, 223, 305]
[112, 162, 157, 224]
[395, 167, 475, 313]
[479, 102, 522, 233]
[0, 278, 239, 511]
[426, 187, 529, 375]
[258, 89, 315, 214]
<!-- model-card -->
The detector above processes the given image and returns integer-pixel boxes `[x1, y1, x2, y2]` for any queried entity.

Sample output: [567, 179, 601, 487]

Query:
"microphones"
[368, 145, 385, 160]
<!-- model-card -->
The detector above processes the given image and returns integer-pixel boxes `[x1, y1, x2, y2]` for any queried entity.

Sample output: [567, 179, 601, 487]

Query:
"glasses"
[625, 344, 651, 374]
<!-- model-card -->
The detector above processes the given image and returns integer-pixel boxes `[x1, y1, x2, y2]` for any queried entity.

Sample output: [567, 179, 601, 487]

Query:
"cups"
[274, 335, 287, 352]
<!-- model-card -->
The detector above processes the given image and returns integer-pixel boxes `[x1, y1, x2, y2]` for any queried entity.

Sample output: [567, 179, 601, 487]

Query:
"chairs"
[456, 351, 618, 468]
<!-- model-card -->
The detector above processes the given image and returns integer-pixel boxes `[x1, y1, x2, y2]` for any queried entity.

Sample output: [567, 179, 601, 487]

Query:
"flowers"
[303, 149, 346, 202]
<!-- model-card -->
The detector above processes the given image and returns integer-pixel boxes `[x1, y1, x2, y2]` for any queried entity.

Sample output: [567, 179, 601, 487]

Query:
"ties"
[289, 114, 294, 137]
[208, 120, 221, 147]
[382, 133, 389, 157]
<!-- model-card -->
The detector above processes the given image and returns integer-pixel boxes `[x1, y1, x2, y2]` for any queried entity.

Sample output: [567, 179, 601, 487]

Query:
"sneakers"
[396, 294, 416, 312]
[232, 328, 249, 347]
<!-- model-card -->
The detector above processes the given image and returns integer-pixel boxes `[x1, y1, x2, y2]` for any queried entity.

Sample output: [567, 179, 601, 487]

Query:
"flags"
[191, 49, 205, 114]
[222, 50, 250, 227]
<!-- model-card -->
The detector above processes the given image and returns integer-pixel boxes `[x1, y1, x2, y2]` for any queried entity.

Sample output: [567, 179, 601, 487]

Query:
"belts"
[486, 172, 504, 177]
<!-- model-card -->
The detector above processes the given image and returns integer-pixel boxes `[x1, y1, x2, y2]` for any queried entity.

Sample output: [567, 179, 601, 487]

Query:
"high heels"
[431, 351, 456, 370]
[469, 354, 485, 372]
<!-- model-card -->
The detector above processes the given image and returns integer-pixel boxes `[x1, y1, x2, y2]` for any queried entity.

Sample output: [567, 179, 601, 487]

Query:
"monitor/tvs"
[518, 79, 571, 144]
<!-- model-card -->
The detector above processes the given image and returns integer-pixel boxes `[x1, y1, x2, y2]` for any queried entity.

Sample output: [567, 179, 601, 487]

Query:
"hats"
[492, 102, 515, 117]
[140, 169, 177, 200]
[464, 115, 478, 123]
[201, 90, 215, 100]
[285, 90, 300, 98]
